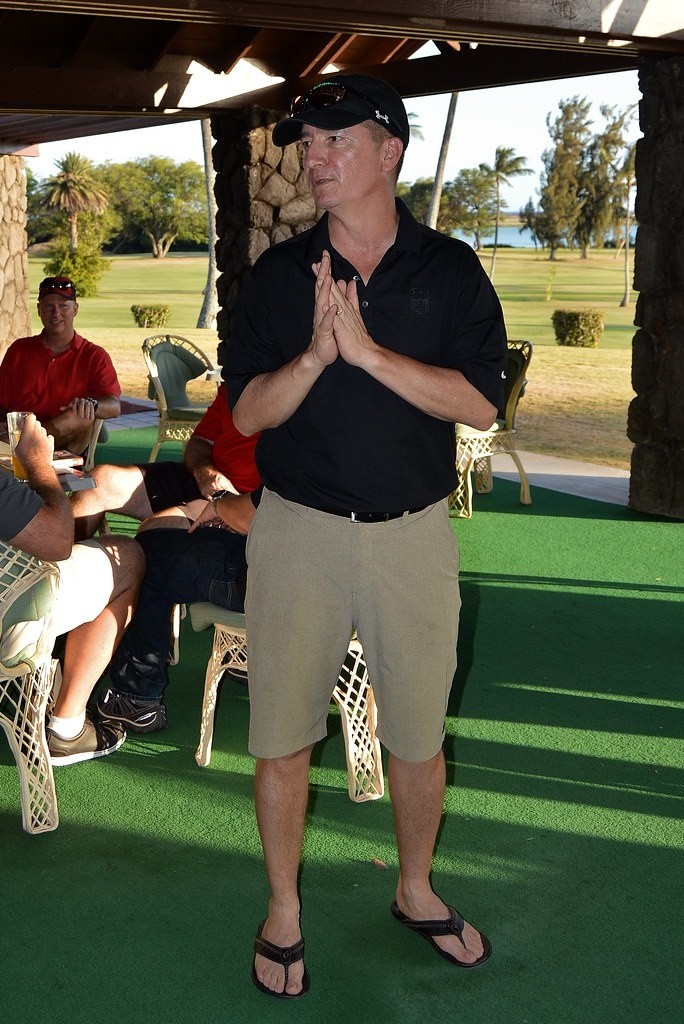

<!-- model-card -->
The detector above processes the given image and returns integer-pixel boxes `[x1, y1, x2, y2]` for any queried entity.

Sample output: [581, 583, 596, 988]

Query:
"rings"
[337, 309, 343, 315]
[84, 403, 89, 406]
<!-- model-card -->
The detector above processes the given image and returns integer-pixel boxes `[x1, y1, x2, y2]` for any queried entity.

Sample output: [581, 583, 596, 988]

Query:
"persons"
[0, 415, 146, 767]
[224, 75, 510, 996]
[0, 276, 122, 470]
[67, 382, 265, 731]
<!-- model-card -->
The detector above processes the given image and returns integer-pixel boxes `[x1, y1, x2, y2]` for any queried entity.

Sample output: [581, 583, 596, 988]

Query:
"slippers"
[251, 919, 309, 1000]
[391, 899, 492, 968]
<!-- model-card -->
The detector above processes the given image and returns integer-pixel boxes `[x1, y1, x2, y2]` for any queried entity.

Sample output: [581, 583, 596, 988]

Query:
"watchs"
[212, 489, 237, 514]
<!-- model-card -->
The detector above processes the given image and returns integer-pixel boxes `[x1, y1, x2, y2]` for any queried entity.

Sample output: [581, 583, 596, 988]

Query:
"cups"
[11, 431, 29, 483]
[7, 411, 34, 443]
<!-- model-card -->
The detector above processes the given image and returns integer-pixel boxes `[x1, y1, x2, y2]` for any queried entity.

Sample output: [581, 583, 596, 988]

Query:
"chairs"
[185, 602, 385, 802]
[81, 418, 112, 537]
[0, 542, 63, 835]
[142, 334, 221, 462]
[448, 339, 532, 519]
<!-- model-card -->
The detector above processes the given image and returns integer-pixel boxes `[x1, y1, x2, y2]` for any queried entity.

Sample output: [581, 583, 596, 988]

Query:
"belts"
[274, 489, 427, 524]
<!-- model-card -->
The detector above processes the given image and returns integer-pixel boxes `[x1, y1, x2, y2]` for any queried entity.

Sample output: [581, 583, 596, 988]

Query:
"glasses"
[39, 281, 75, 290]
[290, 82, 403, 131]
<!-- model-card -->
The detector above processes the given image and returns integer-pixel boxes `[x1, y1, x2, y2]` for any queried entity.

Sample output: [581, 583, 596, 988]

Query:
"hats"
[272, 74, 410, 147]
[37, 277, 76, 301]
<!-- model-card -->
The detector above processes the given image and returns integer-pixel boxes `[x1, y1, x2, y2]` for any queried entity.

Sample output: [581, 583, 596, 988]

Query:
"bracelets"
[85, 396, 98, 412]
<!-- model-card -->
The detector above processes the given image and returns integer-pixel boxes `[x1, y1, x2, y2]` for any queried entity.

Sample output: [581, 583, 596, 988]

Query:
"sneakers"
[96, 689, 166, 734]
[45, 715, 126, 768]
